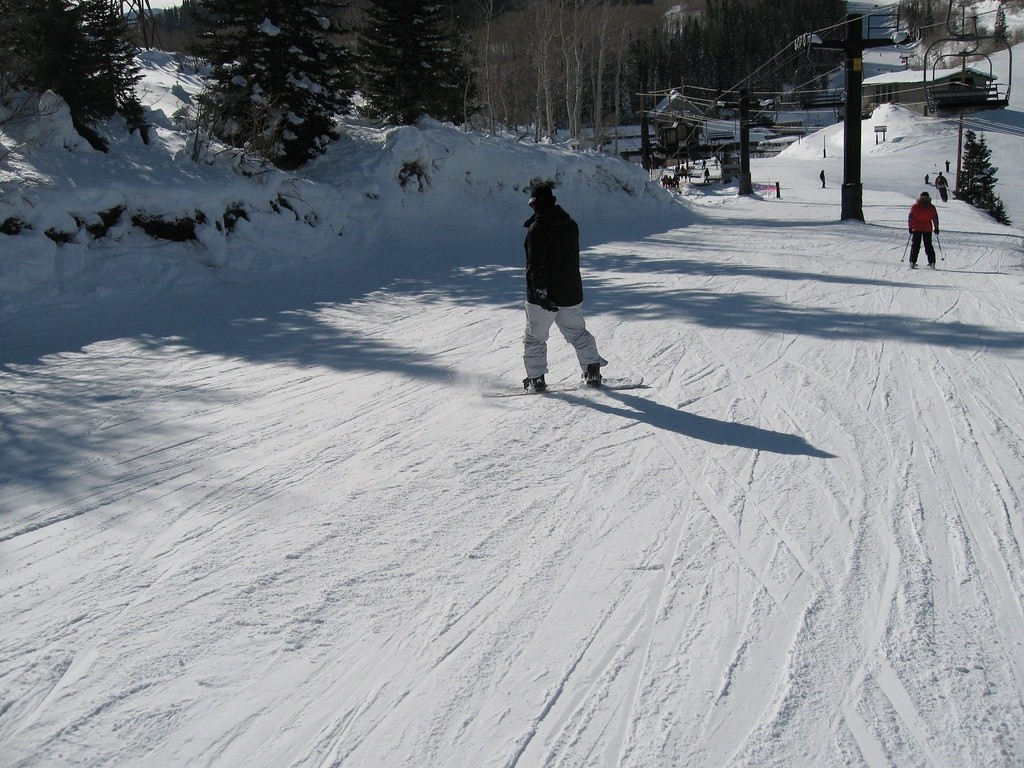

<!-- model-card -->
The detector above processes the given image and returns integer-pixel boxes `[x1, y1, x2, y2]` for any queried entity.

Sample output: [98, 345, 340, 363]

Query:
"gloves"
[537, 292, 559, 312]
[909, 229, 912, 234]
[934, 228, 939, 234]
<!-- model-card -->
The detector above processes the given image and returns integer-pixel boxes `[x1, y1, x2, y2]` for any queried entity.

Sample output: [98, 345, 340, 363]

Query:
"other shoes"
[909, 261, 916, 267]
[929, 262, 935, 269]
[522, 375, 546, 392]
[581, 363, 602, 387]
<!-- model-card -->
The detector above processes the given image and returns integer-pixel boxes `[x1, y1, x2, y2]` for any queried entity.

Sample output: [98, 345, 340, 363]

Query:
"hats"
[530, 184, 556, 204]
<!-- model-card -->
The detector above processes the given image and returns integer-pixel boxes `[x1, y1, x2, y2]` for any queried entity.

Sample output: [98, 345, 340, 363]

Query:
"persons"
[820, 170, 826, 188]
[907, 191, 940, 269]
[662, 159, 719, 189]
[523, 182, 608, 392]
[934, 172, 948, 202]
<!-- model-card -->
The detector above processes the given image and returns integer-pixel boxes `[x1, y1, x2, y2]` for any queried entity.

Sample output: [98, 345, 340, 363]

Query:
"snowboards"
[477, 374, 643, 396]
